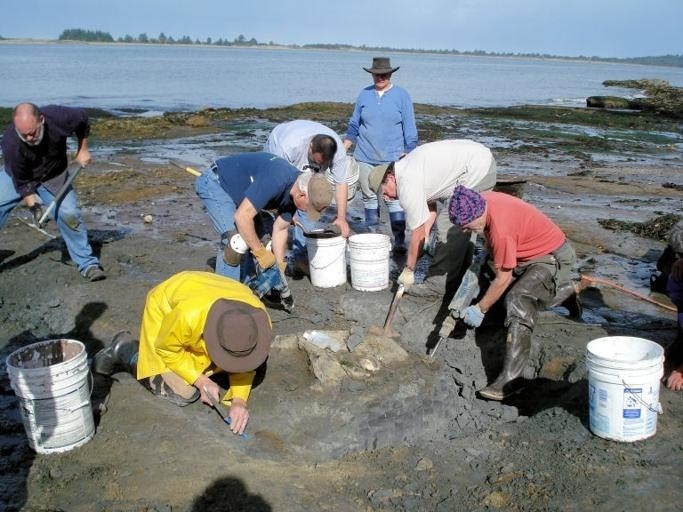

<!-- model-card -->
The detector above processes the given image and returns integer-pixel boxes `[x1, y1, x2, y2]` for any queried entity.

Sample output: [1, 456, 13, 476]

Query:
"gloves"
[278, 262, 287, 275]
[396, 266, 415, 292]
[458, 305, 485, 328]
[28, 203, 50, 229]
[249, 244, 276, 269]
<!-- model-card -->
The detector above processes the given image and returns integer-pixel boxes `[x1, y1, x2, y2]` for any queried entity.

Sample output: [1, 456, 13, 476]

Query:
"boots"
[548, 281, 583, 320]
[91, 329, 152, 392]
[478, 322, 532, 399]
[363, 208, 380, 234]
[389, 211, 407, 254]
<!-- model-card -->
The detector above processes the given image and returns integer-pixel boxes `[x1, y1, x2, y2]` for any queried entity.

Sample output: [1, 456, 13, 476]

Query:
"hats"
[368, 159, 393, 195]
[203, 298, 272, 374]
[360, 56, 399, 76]
[447, 184, 486, 226]
[307, 173, 334, 222]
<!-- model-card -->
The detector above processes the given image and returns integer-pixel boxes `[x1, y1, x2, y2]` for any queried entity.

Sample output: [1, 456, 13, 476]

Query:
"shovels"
[367, 283, 405, 338]
[169, 160, 341, 234]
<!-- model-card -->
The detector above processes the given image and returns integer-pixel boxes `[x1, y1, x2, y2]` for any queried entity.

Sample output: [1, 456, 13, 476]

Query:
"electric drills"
[246, 262, 295, 314]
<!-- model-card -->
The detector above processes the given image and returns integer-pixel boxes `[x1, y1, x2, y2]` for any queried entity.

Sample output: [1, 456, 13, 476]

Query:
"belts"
[209, 161, 220, 179]
[550, 239, 568, 271]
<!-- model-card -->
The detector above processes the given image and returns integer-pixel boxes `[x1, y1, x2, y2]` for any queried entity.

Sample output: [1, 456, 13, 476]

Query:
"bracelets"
[404, 263, 416, 272]
[477, 302, 488, 314]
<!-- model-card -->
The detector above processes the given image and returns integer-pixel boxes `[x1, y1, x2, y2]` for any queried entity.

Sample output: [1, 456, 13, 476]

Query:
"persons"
[342, 56, 419, 260]
[367, 138, 497, 298]
[191, 150, 333, 314]
[0, 102, 104, 281]
[91, 268, 273, 437]
[448, 185, 583, 402]
[658, 218, 682, 394]
[260, 119, 350, 253]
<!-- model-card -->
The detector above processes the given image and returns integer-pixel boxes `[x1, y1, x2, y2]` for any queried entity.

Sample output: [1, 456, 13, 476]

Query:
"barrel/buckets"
[348, 233, 392, 292]
[585, 336, 665, 443]
[305, 228, 347, 288]
[5, 339, 96, 453]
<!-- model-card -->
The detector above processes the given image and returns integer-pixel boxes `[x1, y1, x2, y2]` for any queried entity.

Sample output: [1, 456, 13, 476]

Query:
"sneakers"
[405, 284, 439, 298]
[85, 267, 105, 282]
